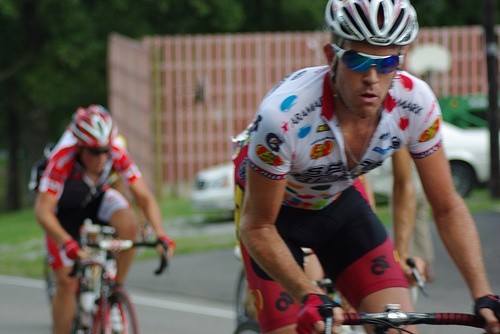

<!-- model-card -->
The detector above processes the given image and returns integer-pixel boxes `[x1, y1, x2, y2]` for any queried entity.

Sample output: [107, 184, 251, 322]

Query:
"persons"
[233, 0, 500, 334]
[35, 105, 176, 334]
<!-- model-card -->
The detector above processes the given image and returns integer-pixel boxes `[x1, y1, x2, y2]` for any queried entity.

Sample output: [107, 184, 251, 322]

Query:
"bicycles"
[233, 246, 486, 334]
[44, 224, 169, 334]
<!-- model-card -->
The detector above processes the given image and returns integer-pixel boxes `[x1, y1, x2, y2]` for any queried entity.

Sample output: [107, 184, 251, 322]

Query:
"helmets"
[324, 0, 420, 46]
[71, 106, 112, 147]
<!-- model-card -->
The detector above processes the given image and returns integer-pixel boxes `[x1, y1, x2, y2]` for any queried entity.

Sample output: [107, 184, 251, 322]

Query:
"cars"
[190, 121, 491, 209]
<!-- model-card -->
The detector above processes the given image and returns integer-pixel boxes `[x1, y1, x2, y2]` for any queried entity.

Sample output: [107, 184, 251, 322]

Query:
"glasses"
[87, 147, 111, 156]
[331, 42, 403, 75]
